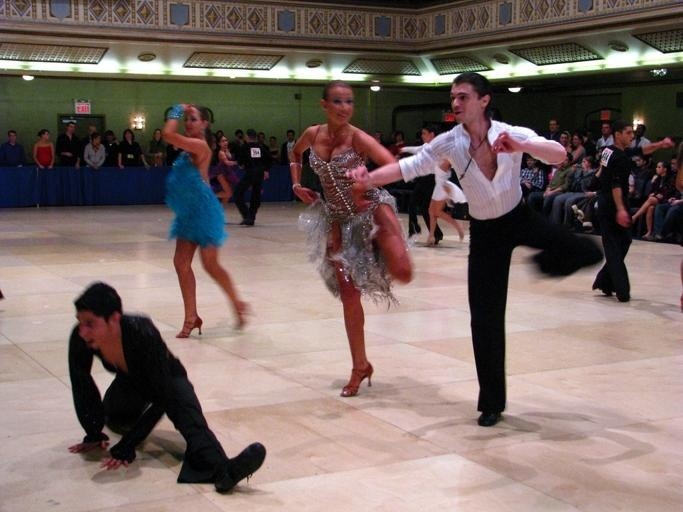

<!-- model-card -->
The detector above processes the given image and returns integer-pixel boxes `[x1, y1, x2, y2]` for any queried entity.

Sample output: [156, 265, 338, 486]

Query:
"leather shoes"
[214, 442, 266, 493]
[478, 411, 501, 426]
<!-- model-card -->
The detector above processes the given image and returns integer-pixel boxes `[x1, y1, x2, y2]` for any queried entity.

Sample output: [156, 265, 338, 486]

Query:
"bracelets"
[290, 181, 302, 192]
[342, 72, 602, 426]
[166, 103, 185, 122]
[289, 161, 304, 170]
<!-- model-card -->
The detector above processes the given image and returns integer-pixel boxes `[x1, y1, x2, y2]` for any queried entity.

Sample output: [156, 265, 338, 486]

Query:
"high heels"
[176, 319, 203, 339]
[342, 363, 373, 397]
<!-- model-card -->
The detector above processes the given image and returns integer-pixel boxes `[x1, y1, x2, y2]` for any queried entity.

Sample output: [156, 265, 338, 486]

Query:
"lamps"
[370, 79, 381, 92]
[129, 114, 146, 130]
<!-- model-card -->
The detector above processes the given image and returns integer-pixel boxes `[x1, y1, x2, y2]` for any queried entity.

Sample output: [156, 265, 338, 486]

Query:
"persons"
[591, 121, 673, 303]
[67, 281, 264, 496]
[287, 82, 412, 396]
[1, 121, 295, 226]
[161, 102, 248, 340]
[371, 119, 683, 246]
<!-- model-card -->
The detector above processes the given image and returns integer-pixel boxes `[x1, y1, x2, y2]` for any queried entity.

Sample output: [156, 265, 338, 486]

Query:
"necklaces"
[458, 133, 486, 182]
